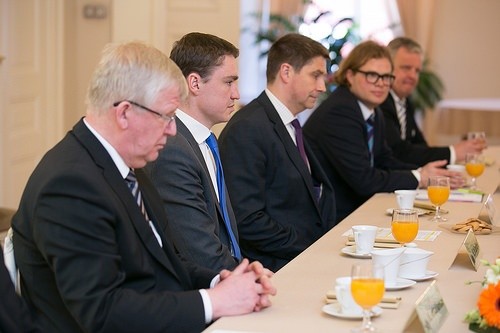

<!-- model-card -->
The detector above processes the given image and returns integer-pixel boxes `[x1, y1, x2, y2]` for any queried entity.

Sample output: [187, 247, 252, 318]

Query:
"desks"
[195, 143, 500, 333]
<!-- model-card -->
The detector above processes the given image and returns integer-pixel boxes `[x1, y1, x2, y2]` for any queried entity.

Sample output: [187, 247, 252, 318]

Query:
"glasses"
[114, 100, 176, 127]
[352, 68, 396, 86]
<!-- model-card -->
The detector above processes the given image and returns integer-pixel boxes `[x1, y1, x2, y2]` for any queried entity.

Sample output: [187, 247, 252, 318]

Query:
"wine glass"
[427, 176, 450, 223]
[392, 208, 419, 247]
[464, 152, 486, 191]
[351, 262, 385, 333]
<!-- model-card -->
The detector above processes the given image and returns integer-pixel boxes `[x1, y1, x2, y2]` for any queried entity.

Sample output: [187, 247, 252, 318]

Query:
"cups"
[466, 131, 486, 139]
[335, 276, 364, 314]
[446, 164, 465, 172]
[352, 225, 379, 255]
[369, 247, 433, 286]
[395, 190, 415, 210]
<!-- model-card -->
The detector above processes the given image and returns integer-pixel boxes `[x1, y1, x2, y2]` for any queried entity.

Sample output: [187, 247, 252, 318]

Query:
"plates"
[398, 270, 440, 281]
[384, 279, 417, 290]
[385, 207, 428, 217]
[340, 246, 377, 259]
[322, 303, 383, 319]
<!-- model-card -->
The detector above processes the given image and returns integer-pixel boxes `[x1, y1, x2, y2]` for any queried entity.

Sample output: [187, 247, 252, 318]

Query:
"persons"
[302, 40, 468, 215]
[218, 33, 337, 273]
[10, 41, 277, 333]
[139, 32, 273, 279]
[380, 37, 488, 167]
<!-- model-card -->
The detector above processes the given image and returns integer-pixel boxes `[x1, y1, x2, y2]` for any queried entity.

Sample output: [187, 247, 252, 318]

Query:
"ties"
[125, 171, 160, 247]
[207, 132, 241, 260]
[291, 119, 311, 175]
[365, 114, 374, 165]
[397, 101, 406, 139]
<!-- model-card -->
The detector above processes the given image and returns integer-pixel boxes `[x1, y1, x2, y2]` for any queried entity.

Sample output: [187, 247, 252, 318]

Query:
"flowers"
[463, 258, 500, 333]
